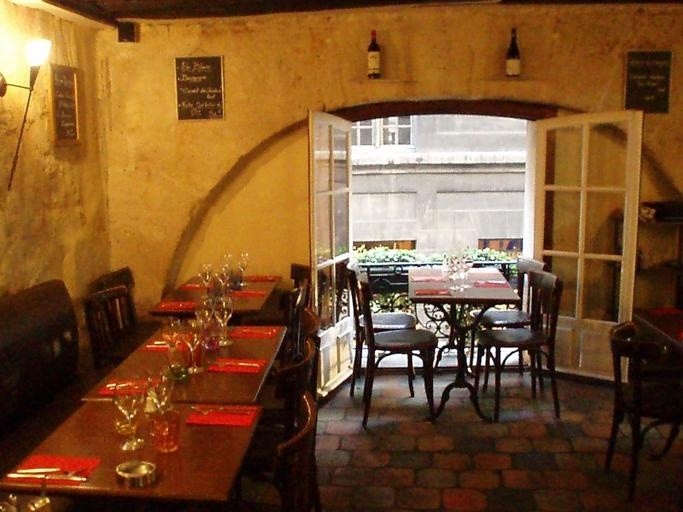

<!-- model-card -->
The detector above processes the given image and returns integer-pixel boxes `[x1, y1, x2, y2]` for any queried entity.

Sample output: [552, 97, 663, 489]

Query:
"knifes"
[6, 472, 87, 483]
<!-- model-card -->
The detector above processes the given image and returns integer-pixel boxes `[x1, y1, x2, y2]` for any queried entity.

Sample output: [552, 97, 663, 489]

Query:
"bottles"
[504, 27, 522, 77]
[367, 29, 381, 79]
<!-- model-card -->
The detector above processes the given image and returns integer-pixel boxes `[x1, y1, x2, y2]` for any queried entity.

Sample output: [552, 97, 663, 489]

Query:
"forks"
[17, 464, 84, 476]
[190, 406, 249, 417]
[208, 358, 258, 368]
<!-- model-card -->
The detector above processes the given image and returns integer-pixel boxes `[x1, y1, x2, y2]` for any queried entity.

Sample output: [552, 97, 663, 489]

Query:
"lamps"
[5, 38, 51, 190]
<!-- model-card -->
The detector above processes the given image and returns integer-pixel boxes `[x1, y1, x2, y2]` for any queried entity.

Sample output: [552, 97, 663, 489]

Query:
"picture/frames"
[626, 48, 672, 113]
[51, 60, 86, 147]
[175, 56, 226, 121]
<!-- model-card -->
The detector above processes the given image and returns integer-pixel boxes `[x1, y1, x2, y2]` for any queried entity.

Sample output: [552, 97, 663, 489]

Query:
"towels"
[474, 279, 509, 289]
[185, 404, 259, 427]
[98, 376, 162, 396]
[4, 454, 103, 485]
[180, 282, 204, 290]
[229, 325, 278, 339]
[208, 356, 266, 375]
[246, 274, 281, 282]
[413, 287, 452, 296]
[410, 276, 447, 283]
[646, 305, 681, 317]
[151, 300, 195, 310]
[227, 289, 262, 300]
[142, 335, 189, 353]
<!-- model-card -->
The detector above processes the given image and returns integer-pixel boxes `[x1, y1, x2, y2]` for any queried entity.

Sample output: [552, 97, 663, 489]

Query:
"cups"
[456, 262, 467, 292]
[183, 321, 205, 375]
[147, 411, 181, 452]
[465, 257, 474, 282]
[237, 254, 249, 287]
[160, 316, 179, 347]
[199, 263, 214, 286]
[114, 384, 147, 450]
[210, 299, 234, 347]
[137, 360, 171, 415]
[216, 265, 230, 290]
[192, 293, 214, 328]
[221, 253, 233, 269]
[447, 265, 459, 290]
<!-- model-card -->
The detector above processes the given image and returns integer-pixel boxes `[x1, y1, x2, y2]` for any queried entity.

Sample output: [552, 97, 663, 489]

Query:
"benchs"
[0, 278, 115, 482]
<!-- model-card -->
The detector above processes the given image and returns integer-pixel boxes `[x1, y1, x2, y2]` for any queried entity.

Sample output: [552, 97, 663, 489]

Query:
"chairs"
[607, 321, 683, 500]
[255, 339, 320, 441]
[239, 393, 320, 511]
[82, 284, 160, 374]
[244, 276, 307, 324]
[360, 278, 438, 427]
[476, 255, 546, 396]
[92, 265, 158, 331]
[279, 309, 320, 355]
[476, 269, 564, 423]
[346, 258, 415, 400]
